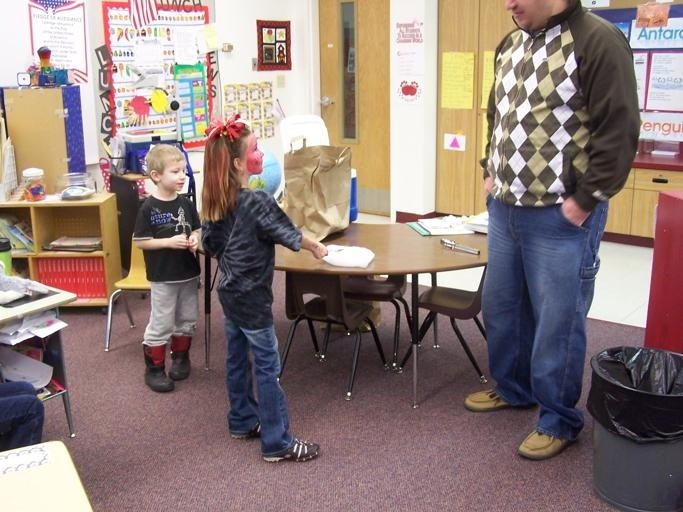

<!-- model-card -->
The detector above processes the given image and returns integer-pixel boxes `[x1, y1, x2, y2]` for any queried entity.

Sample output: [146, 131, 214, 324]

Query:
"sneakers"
[232, 422, 260, 439]
[464, 387, 537, 412]
[262, 437, 321, 464]
[518, 430, 577, 460]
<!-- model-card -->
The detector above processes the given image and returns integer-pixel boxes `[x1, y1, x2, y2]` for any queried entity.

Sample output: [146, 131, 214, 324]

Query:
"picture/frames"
[256, 20, 294, 72]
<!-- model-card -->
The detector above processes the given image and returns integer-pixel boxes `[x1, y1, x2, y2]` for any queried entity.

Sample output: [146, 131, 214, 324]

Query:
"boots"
[168, 336, 192, 381]
[143, 343, 175, 392]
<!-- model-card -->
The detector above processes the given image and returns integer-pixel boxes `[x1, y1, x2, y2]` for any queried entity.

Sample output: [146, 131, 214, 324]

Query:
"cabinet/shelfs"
[603, 159, 634, 244]
[438, 0, 517, 216]
[1, 193, 123, 314]
[0, 85, 87, 191]
[631, 168, 683, 245]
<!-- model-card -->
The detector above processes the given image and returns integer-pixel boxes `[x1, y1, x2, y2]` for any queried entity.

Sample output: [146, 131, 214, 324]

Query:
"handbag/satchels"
[283, 135, 352, 241]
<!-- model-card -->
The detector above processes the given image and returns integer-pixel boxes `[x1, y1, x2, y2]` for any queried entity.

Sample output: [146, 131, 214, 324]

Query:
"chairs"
[277, 270, 390, 402]
[104, 233, 153, 353]
[342, 275, 418, 370]
[400, 265, 495, 384]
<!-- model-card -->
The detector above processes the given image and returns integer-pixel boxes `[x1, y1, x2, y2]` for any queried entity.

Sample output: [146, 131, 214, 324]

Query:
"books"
[0, 211, 33, 253]
[405, 212, 489, 236]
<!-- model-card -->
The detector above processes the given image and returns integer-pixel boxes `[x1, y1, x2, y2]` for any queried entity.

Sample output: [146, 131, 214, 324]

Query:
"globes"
[247, 143, 282, 195]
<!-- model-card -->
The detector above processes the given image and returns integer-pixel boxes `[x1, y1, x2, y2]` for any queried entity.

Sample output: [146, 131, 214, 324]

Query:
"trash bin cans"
[591, 346, 683, 508]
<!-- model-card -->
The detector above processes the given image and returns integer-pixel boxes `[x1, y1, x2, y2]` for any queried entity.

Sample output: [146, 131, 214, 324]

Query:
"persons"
[466, 0, 641, 460]
[134, 145, 202, 392]
[0, 381, 44, 451]
[201, 114, 329, 466]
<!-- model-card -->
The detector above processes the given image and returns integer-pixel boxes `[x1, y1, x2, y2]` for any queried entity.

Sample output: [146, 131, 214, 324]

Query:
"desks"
[190, 212, 489, 410]
[0, 275, 78, 438]
